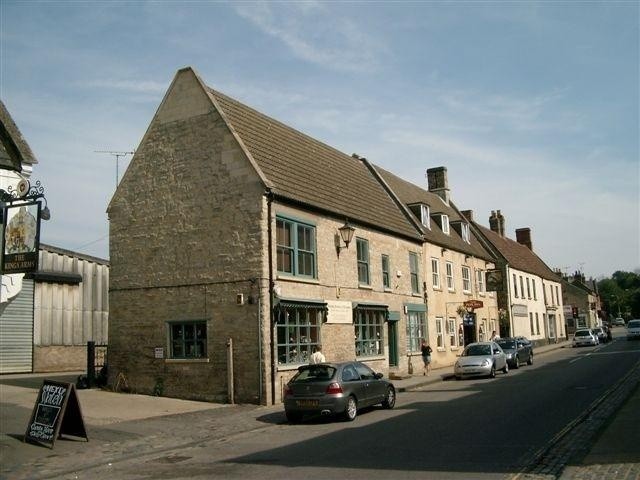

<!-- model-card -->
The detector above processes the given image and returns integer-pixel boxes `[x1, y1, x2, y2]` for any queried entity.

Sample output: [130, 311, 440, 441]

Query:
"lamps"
[337, 218, 355, 256]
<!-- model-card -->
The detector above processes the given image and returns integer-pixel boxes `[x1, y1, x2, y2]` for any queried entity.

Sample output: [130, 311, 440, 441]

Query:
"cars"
[452, 342, 508, 379]
[571, 318, 640, 348]
[282, 360, 397, 421]
[496, 336, 535, 370]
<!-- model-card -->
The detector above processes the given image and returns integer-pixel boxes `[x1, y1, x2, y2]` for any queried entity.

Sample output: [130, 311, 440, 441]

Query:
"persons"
[490, 331, 498, 343]
[421, 338, 432, 374]
[309, 344, 325, 365]
